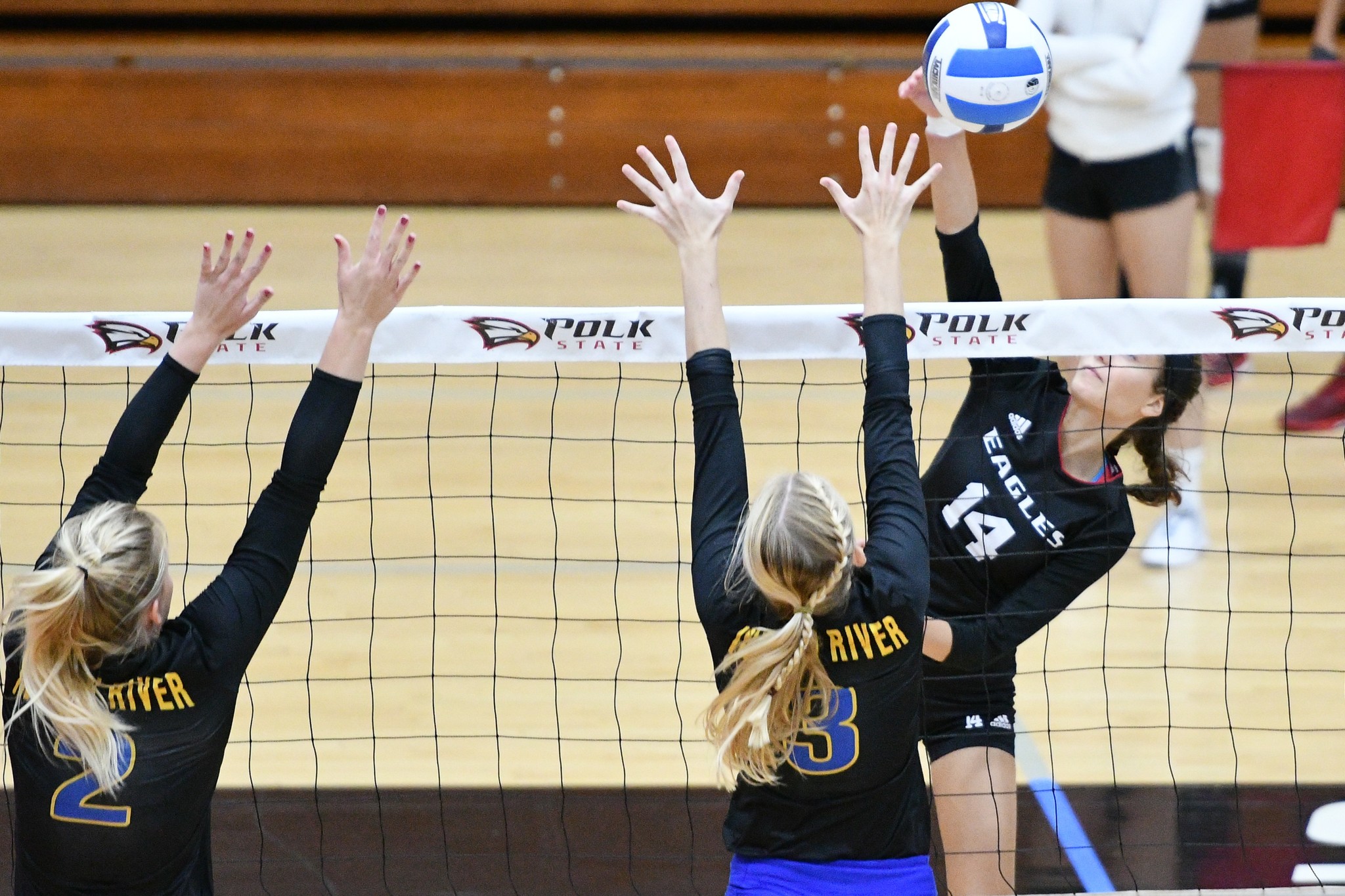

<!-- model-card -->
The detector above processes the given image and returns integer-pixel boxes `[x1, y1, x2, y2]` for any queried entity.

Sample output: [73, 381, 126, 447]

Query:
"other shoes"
[1204, 352, 1252, 391]
[1310, 47, 1338, 61]
[1278, 357, 1344, 435]
[1142, 510, 1212, 566]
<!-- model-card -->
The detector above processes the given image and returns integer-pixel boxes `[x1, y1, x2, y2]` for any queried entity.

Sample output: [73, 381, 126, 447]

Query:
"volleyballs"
[922, 2, 1053, 134]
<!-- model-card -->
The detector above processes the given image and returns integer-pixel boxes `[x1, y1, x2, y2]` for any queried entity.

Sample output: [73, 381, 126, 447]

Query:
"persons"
[614, 122, 940, 896]
[1010, 0, 1259, 562]
[0, 202, 420, 896]
[1280, 1, 1345, 433]
[898, 53, 1206, 896]
